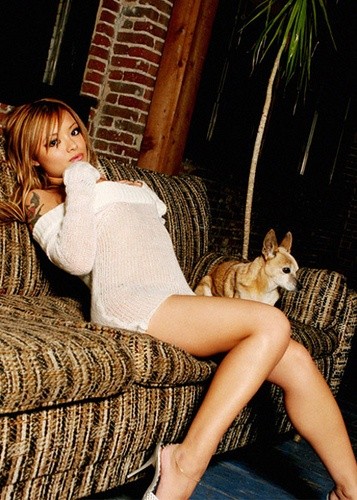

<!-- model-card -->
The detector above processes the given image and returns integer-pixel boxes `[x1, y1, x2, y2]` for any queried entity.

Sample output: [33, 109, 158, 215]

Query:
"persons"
[0, 98, 357, 500]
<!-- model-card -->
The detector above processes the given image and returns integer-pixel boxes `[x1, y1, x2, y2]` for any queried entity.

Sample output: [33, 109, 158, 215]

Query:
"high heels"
[127, 443, 163, 500]
[326, 488, 348, 500]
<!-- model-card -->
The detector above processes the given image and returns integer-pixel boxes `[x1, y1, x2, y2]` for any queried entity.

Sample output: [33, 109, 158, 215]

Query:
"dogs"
[194, 228, 303, 305]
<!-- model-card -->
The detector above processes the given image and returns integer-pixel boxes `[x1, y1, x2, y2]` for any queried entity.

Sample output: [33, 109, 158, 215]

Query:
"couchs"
[0, 136, 357, 500]
